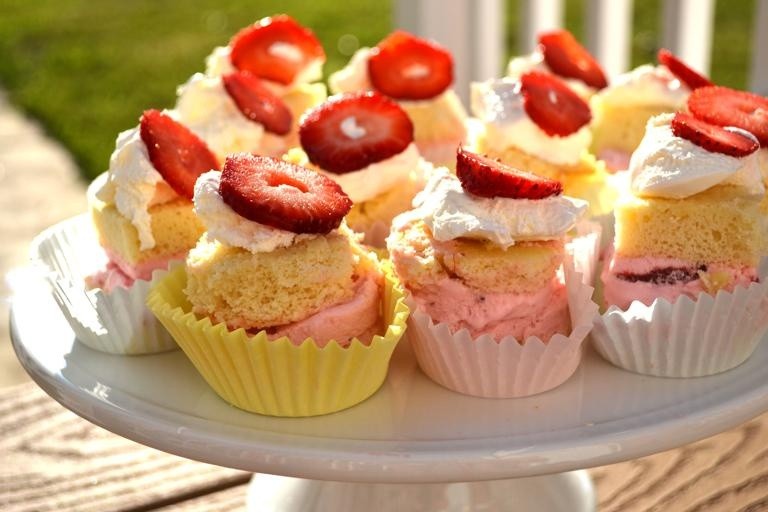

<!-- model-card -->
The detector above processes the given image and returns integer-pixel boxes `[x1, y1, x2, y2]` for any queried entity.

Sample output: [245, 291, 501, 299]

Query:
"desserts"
[87, 12, 767, 419]
